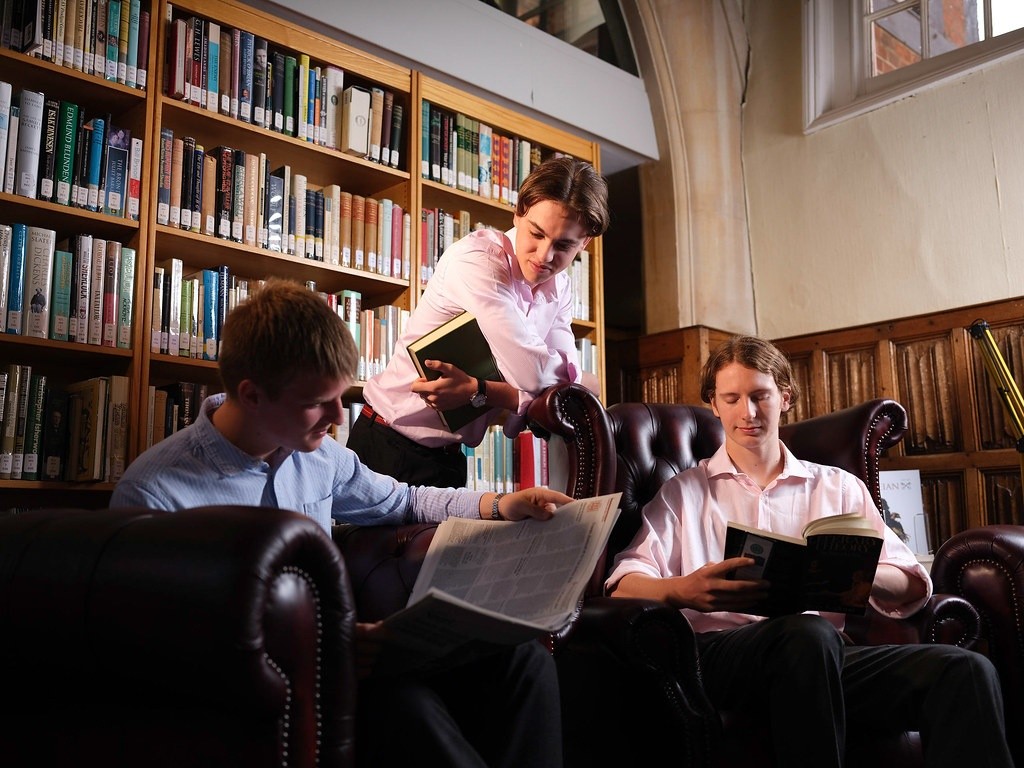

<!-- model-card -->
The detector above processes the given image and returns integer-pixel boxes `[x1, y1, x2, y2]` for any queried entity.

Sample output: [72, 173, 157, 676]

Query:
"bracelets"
[491, 493, 509, 521]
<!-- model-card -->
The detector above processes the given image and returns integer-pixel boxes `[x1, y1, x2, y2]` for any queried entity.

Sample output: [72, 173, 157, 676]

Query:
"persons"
[346, 157, 610, 486]
[108, 279, 577, 768]
[117, 130, 127, 145]
[108, 132, 120, 148]
[254, 47, 266, 70]
[241, 90, 250, 103]
[600, 335, 1014, 768]
[30, 287, 46, 313]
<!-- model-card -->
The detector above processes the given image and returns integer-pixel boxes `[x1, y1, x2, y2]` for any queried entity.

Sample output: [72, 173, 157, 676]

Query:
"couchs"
[0, 381, 1024, 768]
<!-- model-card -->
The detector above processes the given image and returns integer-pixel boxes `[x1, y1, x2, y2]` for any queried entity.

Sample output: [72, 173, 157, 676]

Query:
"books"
[406, 310, 508, 434]
[0, 0, 597, 483]
[381, 492, 623, 676]
[724, 511, 884, 619]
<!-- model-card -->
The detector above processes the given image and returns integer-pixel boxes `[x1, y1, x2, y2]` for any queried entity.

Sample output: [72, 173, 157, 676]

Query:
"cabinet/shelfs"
[0, 0, 614, 511]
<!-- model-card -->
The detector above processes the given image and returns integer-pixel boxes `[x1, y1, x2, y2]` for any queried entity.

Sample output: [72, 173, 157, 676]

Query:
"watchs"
[469, 377, 487, 408]
